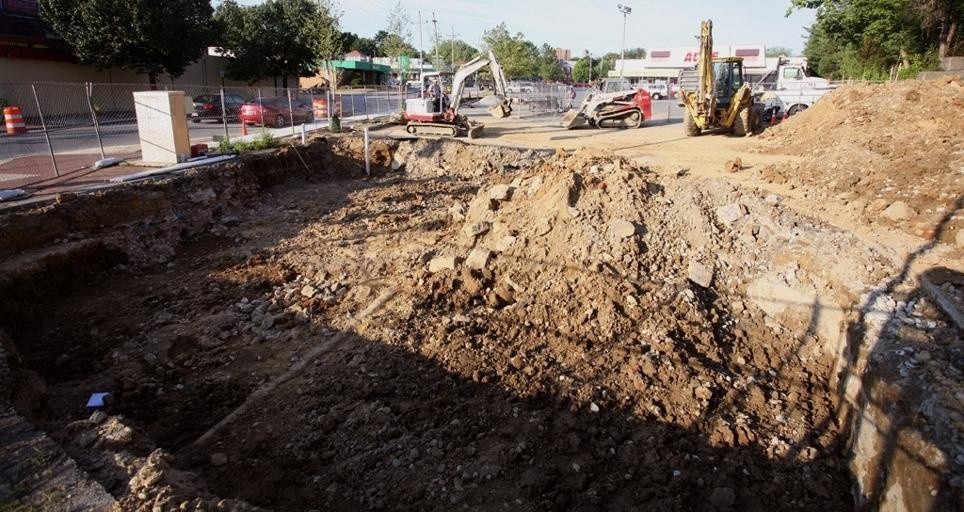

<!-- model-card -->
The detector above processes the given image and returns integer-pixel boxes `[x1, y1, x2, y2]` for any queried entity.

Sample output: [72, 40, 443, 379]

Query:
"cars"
[240, 97, 314, 128]
[190, 95, 245, 123]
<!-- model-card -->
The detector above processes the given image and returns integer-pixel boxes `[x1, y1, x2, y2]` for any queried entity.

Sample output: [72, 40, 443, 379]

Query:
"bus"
[632, 84, 668, 100]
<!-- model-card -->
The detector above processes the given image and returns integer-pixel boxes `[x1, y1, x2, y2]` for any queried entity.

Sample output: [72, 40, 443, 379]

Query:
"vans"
[505, 82, 534, 94]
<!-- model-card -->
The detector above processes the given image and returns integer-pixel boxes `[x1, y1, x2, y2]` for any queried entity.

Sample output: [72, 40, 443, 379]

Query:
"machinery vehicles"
[677, 19, 842, 136]
[560, 77, 651, 129]
[402, 49, 513, 138]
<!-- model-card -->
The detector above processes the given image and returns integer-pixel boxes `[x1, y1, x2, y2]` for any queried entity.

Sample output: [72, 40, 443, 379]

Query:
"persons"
[427, 79, 440, 98]
[567, 85, 576, 106]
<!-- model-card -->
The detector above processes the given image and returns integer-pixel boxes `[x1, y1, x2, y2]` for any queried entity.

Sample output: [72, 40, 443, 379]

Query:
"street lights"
[618, 4, 631, 78]
[585, 50, 592, 85]
